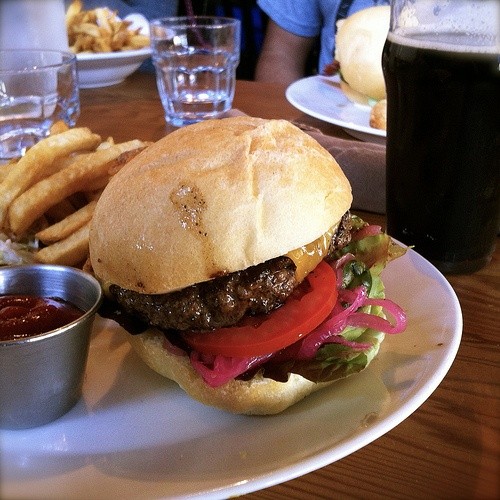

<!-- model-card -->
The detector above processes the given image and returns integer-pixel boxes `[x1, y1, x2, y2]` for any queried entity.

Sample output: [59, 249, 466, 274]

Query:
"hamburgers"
[83, 114, 407, 416]
[334, 6, 390, 130]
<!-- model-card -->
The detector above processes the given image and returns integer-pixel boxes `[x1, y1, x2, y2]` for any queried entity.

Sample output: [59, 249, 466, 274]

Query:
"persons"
[254, 0, 393, 83]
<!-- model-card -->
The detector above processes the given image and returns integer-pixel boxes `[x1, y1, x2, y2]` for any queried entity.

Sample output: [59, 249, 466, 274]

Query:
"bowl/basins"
[77, 25, 177, 90]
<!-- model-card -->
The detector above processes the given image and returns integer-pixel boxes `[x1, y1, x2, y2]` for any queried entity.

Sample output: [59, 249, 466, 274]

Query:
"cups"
[149, 14, 243, 127]
[381, 0, 500, 276]
[0, 47, 81, 160]
[0, 263, 104, 431]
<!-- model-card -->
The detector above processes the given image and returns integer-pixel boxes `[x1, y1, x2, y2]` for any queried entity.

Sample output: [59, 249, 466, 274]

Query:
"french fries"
[65, 0, 151, 54]
[0, 121, 146, 268]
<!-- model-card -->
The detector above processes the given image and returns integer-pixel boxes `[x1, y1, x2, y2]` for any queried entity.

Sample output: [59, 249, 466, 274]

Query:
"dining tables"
[0, 69, 500, 500]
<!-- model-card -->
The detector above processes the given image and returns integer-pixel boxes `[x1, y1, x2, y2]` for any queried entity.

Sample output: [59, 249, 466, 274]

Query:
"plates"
[285, 71, 387, 138]
[0, 232, 464, 500]
